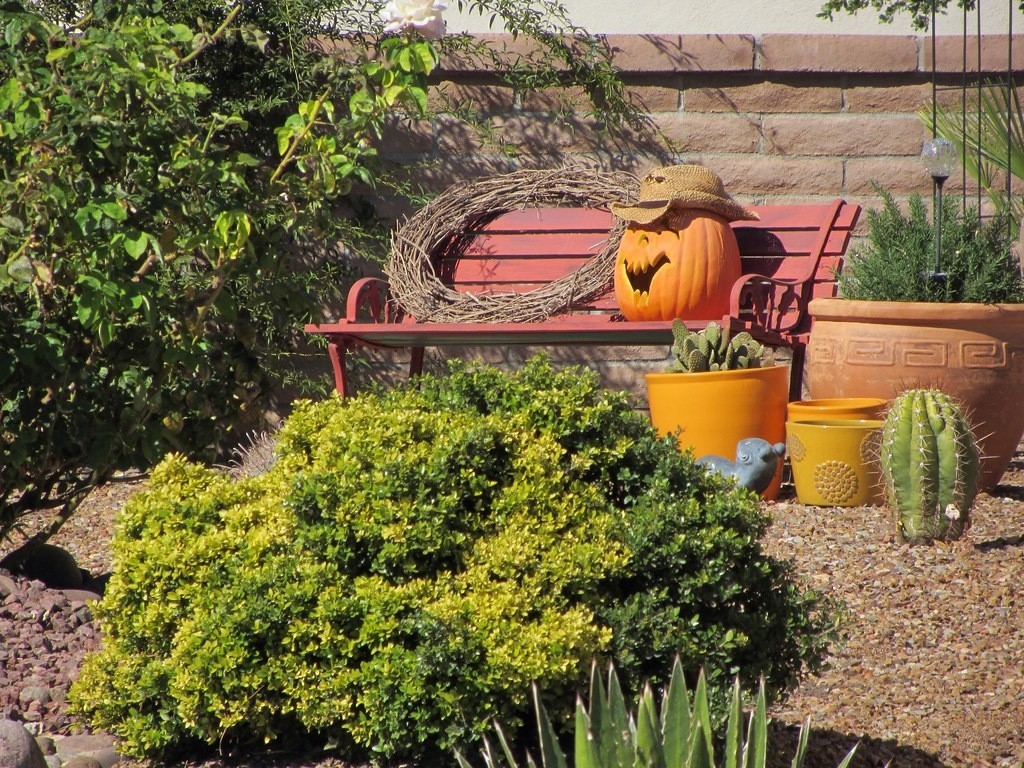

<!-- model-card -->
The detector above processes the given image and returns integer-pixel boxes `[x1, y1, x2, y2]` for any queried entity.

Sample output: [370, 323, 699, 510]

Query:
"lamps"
[920, 139, 961, 301]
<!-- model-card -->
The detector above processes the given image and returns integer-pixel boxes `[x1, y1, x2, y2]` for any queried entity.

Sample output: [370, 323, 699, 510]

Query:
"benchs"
[304, 198, 862, 403]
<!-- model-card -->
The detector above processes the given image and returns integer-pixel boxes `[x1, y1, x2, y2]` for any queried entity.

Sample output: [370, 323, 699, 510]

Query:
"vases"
[785, 397, 890, 506]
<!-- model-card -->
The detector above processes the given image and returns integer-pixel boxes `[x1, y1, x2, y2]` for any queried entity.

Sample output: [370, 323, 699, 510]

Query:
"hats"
[612, 164, 761, 224]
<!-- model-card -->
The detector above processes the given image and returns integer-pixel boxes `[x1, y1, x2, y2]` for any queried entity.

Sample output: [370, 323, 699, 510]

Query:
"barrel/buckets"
[786, 419, 887, 506]
[789, 398, 887, 421]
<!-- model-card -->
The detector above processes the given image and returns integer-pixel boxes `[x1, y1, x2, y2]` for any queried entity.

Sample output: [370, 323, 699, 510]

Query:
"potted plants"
[805, 178, 1024, 492]
[645, 319, 790, 500]
